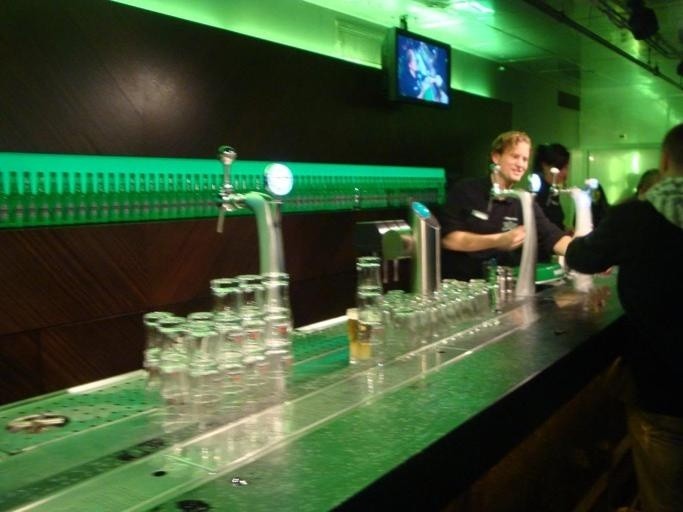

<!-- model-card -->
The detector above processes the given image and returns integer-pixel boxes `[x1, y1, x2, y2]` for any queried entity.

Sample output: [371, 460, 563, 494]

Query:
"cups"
[343, 255, 391, 367]
[388, 276, 489, 363]
[139, 272, 294, 406]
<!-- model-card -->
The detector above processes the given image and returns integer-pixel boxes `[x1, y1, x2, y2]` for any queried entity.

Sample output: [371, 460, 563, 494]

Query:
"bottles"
[0, 170, 441, 228]
[483, 257, 512, 310]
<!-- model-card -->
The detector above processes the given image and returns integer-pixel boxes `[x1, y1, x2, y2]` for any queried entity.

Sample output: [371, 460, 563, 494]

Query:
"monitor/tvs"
[382, 27, 453, 108]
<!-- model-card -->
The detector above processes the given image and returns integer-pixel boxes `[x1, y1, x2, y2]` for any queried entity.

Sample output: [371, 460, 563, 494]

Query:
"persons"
[565, 122, 683, 511]
[434, 130, 571, 280]
[588, 180, 611, 229]
[625, 167, 663, 207]
[402, 42, 447, 103]
[529, 140, 573, 266]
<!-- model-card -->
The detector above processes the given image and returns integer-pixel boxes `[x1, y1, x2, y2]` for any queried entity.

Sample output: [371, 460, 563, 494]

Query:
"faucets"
[546, 166, 594, 238]
[486, 163, 539, 297]
[212, 143, 284, 312]
